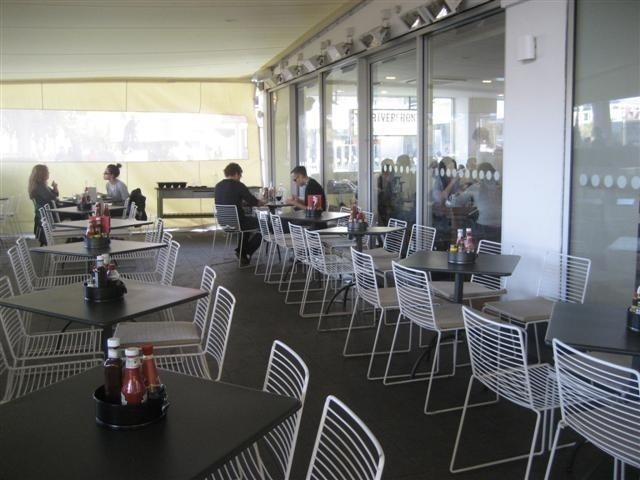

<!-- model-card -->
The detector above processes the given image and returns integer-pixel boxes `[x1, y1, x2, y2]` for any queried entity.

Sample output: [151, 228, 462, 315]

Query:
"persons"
[96, 163, 129, 216]
[377, 127, 502, 239]
[285, 166, 325, 212]
[28, 164, 59, 224]
[216, 163, 263, 266]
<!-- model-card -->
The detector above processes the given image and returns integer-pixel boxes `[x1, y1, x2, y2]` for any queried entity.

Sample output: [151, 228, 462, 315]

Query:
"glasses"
[294, 175, 299, 182]
[104, 172, 112, 176]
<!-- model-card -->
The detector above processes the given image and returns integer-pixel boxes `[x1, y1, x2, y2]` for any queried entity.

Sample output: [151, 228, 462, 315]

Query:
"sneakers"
[235, 254, 249, 267]
[235, 247, 239, 254]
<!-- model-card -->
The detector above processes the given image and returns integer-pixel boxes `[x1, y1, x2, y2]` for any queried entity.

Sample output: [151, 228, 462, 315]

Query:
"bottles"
[121, 347, 147, 405]
[450, 227, 475, 253]
[86, 201, 111, 238]
[141, 343, 162, 400]
[103, 337, 123, 402]
[95, 253, 120, 287]
